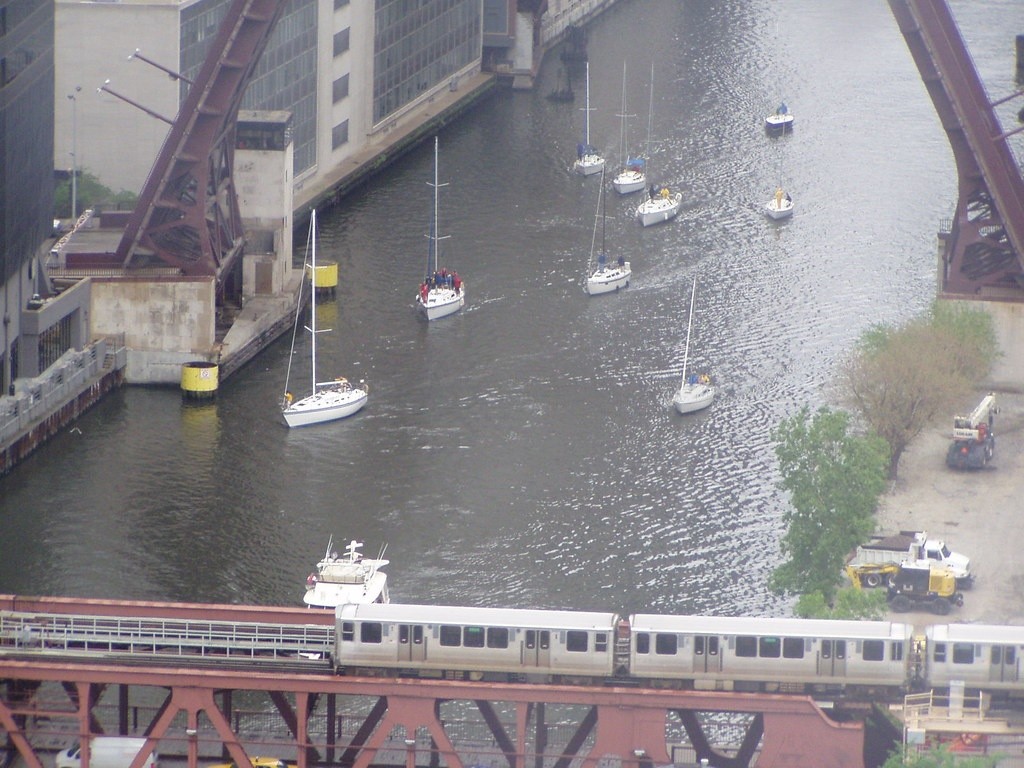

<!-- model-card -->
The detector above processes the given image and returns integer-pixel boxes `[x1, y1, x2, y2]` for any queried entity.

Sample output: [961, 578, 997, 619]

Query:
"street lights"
[66, 86, 82, 225]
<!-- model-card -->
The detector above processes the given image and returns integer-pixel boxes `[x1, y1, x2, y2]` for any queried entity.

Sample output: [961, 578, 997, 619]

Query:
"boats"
[296, 536, 393, 659]
[764, 114, 795, 138]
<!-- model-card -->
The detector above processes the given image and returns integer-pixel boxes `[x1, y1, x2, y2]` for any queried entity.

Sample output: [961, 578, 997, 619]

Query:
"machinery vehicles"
[847, 560, 964, 615]
[946, 390, 1000, 470]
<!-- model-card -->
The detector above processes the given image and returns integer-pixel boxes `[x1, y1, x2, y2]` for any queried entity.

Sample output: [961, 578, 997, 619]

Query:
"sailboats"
[671, 274, 715, 414]
[575, 60, 606, 176]
[635, 64, 683, 227]
[583, 161, 632, 295]
[415, 134, 464, 321]
[765, 121, 794, 219]
[281, 209, 369, 427]
[613, 60, 646, 195]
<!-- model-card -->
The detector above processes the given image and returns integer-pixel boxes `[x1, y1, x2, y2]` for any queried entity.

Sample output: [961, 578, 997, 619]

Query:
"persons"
[776, 189, 783, 209]
[421, 265, 461, 302]
[618, 254, 625, 272]
[576, 142, 584, 162]
[661, 186, 673, 205]
[786, 193, 792, 206]
[598, 252, 606, 272]
[333, 375, 348, 393]
[775, 109, 780, 120]
[690, 372, 710, 380]
[285, 390, 293, 410]
[649, 184, 655, 204]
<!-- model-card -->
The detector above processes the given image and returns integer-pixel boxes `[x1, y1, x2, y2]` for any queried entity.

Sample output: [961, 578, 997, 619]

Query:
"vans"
[56, 735, 156, 768]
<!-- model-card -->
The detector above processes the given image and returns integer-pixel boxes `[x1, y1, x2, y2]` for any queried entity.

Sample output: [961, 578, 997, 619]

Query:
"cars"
[204, 757, 300, 768]
[49, 219, 61, 238]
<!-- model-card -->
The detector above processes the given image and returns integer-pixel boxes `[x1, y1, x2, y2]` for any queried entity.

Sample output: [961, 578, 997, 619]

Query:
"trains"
[327, 602, 1022, 697]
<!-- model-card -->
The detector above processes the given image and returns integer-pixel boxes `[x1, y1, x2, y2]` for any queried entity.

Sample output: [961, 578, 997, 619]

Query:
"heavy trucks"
[855, 529, 972, 587]
